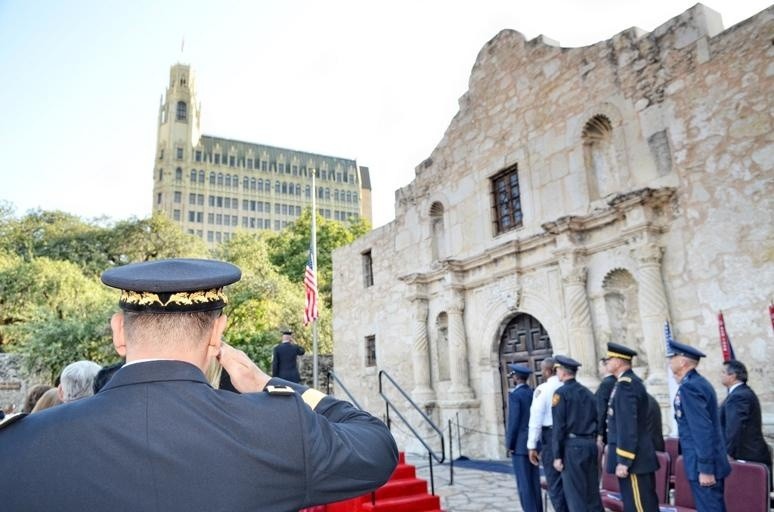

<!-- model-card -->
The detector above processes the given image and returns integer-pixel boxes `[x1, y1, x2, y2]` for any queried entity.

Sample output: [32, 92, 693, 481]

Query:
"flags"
[303, 245, 319, 325]
[663, 320, 674, 356]
[717, 310, 734, 361]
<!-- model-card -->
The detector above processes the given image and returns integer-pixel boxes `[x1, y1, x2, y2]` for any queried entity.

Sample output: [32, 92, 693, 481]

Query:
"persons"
[0, 360, 125, 422]
[503, 342, 665, 512]
[717, 359, 772, 486]
[270, 329, 305, 382]
[667, 339, 732, 511]
[0, 257, 398, 511]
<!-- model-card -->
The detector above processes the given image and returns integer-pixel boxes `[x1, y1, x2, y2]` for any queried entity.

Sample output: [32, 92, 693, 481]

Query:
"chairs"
[534, 434, 774, 512]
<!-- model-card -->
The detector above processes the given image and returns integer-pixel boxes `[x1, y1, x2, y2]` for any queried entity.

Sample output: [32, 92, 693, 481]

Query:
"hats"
[551, 354, 583, 370]
[507, 363, 533, 378]
[600, 341, 638, 361]
[664, 339, 706, 361]
[100, 258, 243, 313]
